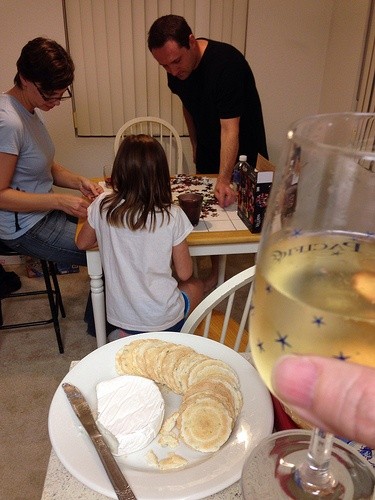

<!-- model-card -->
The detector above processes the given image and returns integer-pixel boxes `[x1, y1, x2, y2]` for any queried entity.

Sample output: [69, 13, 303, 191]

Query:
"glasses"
[32, 82, 73, 103]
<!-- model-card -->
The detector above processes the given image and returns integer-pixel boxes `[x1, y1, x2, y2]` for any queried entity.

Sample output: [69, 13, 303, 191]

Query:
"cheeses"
[96, 374, 165, 455]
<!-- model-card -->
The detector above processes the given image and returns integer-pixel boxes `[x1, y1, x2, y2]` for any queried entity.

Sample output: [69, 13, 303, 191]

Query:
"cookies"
[115, 338, 243, 467]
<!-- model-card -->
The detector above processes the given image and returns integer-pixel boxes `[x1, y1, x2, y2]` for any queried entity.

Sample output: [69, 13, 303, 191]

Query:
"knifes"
[62, 382, 137, 500]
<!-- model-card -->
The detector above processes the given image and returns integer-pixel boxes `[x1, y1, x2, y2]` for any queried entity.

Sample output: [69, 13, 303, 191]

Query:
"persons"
[148, 15, 269, 292]
[0, 38, 117, 338]
[270, 273, 375, 449]
[77, 135, 204, 342]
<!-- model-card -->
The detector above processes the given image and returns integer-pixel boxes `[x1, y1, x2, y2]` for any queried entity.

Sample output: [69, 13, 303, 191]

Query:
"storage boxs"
[236, 153, 276, 234]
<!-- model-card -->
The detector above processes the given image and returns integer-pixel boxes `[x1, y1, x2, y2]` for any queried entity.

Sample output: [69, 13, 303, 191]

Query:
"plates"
[47, 330, 275, 500]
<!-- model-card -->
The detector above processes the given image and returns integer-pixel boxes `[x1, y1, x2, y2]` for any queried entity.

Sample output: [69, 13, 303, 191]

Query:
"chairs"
[114, 117, 184, 177]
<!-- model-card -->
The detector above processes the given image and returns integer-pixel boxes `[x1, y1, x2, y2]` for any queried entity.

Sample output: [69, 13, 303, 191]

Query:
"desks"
[39, 354, 375, 500]
[73, 175, 261, 349]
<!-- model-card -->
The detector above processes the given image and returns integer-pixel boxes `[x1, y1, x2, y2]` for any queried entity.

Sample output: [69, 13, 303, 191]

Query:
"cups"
[177, 193, 204, 226]
[103, 165, 114, 188]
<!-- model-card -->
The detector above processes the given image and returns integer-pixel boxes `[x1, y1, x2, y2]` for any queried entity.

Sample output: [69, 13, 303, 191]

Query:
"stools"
[180, 264, 257, 353]
[0, 241, 66, 355]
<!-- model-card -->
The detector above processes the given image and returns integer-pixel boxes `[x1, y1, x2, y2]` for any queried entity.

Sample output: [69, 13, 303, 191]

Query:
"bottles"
[232, 155, 247, 211]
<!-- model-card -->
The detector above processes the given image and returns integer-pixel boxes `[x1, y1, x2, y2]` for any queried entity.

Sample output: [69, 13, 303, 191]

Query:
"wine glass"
[242, 108, 374, 500]
